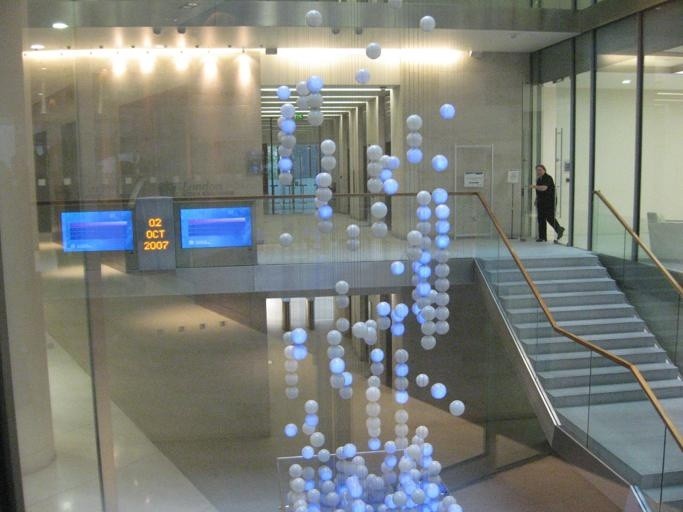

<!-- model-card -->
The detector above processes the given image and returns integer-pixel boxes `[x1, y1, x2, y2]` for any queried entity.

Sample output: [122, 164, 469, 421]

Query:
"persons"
[529, 164, 565, 242]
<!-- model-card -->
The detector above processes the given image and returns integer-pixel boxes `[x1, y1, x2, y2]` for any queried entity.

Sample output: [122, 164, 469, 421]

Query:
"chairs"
[647, 212, 683, 261]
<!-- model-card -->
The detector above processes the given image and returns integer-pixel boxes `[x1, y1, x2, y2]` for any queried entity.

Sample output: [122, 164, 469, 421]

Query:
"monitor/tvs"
[174, 199, 257, 268]
